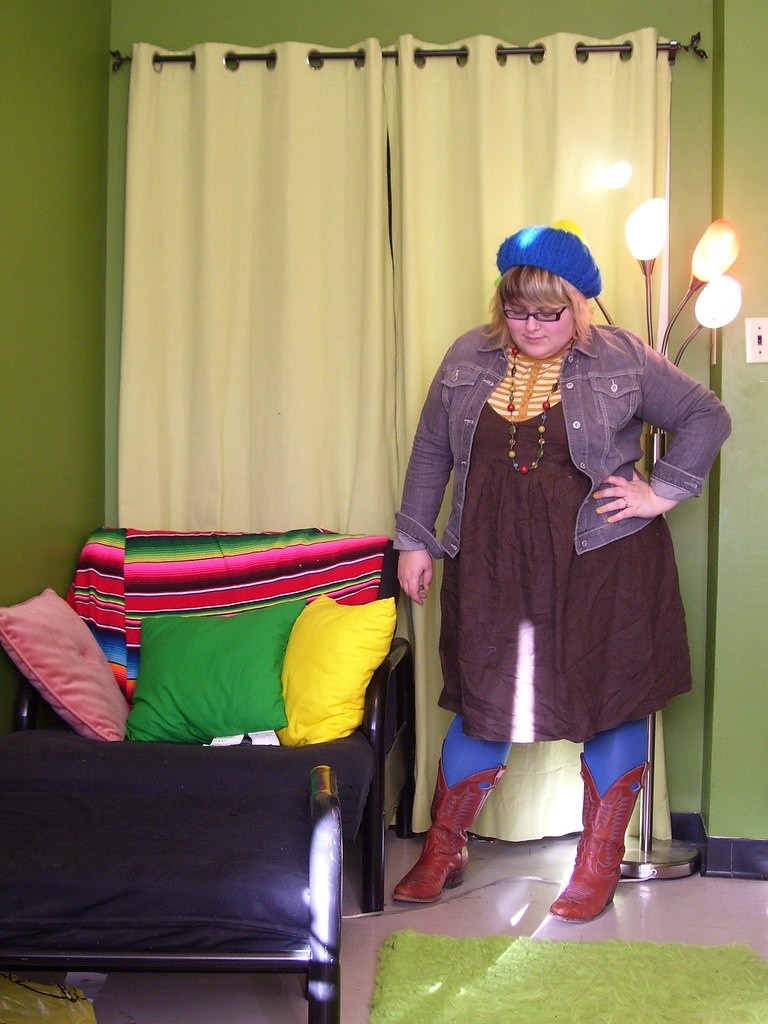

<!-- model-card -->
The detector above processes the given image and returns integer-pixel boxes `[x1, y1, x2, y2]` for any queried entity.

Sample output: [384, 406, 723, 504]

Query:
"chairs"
[0, 527, 415, 1024]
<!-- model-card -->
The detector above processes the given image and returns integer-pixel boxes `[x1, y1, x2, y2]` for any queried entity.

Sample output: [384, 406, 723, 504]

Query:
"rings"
[622, 498, 628, 507]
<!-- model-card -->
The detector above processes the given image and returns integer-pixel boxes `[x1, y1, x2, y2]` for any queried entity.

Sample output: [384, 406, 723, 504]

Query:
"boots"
[392, 740, 506, 904]
[549, 752, 650, 923]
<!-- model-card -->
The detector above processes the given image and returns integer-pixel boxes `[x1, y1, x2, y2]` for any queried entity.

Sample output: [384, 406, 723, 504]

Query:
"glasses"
[501, 300, 566, 322]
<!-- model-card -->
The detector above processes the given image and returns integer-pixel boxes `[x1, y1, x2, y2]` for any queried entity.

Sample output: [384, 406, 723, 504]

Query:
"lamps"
[594, 198, 741, 879]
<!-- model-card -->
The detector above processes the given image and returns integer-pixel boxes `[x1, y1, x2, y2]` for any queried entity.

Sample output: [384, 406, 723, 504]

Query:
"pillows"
[1, 589, 131, 742]
[276, 594, 398, 746]
[125, 599, 309, 740]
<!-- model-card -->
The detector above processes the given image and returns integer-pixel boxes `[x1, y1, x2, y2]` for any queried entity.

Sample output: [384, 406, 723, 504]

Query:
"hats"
[495, 219, 602, 298]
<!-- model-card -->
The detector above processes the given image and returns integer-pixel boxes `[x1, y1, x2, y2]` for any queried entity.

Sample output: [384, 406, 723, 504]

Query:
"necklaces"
[507, 340, 575, 473]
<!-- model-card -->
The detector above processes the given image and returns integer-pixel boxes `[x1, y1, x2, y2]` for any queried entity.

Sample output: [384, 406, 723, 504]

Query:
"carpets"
[366, 929, 768, 1023]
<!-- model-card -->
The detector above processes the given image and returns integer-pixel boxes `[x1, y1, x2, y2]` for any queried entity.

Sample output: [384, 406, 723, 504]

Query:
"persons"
[391, 226, 734, 925]
